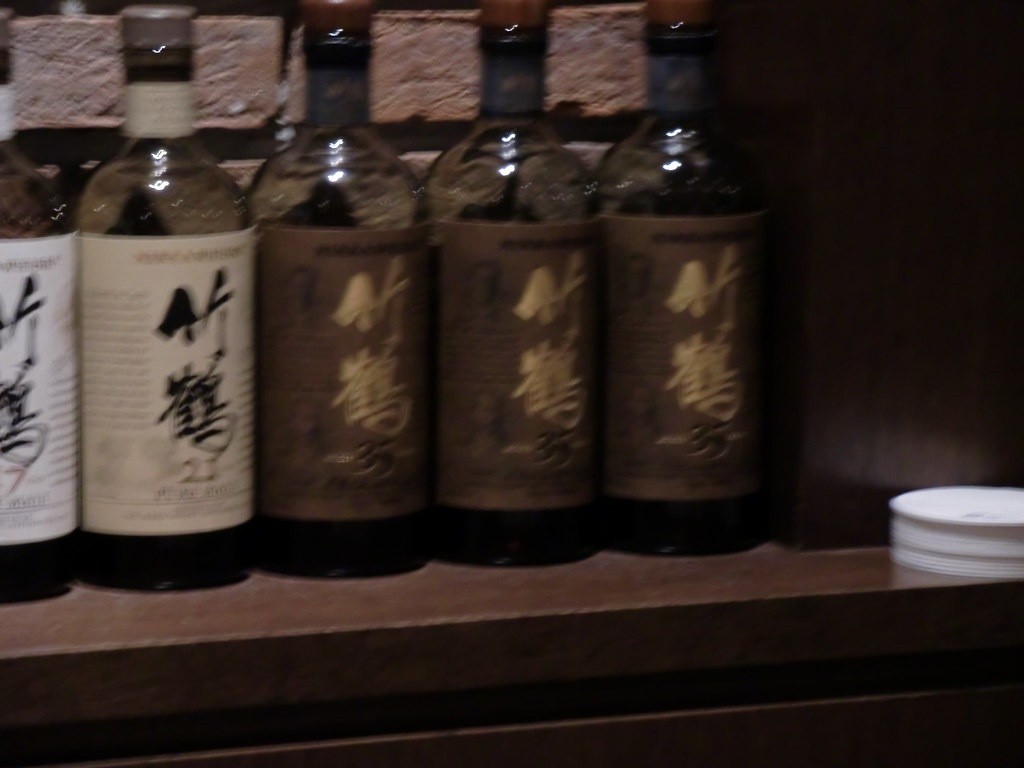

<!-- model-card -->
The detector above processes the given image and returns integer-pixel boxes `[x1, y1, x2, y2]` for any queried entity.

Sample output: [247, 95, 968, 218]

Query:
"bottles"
[0, 1, 773, 606]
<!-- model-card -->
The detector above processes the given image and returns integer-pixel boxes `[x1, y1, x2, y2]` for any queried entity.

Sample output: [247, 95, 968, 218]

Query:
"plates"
[888, 485, 1024, 579]
